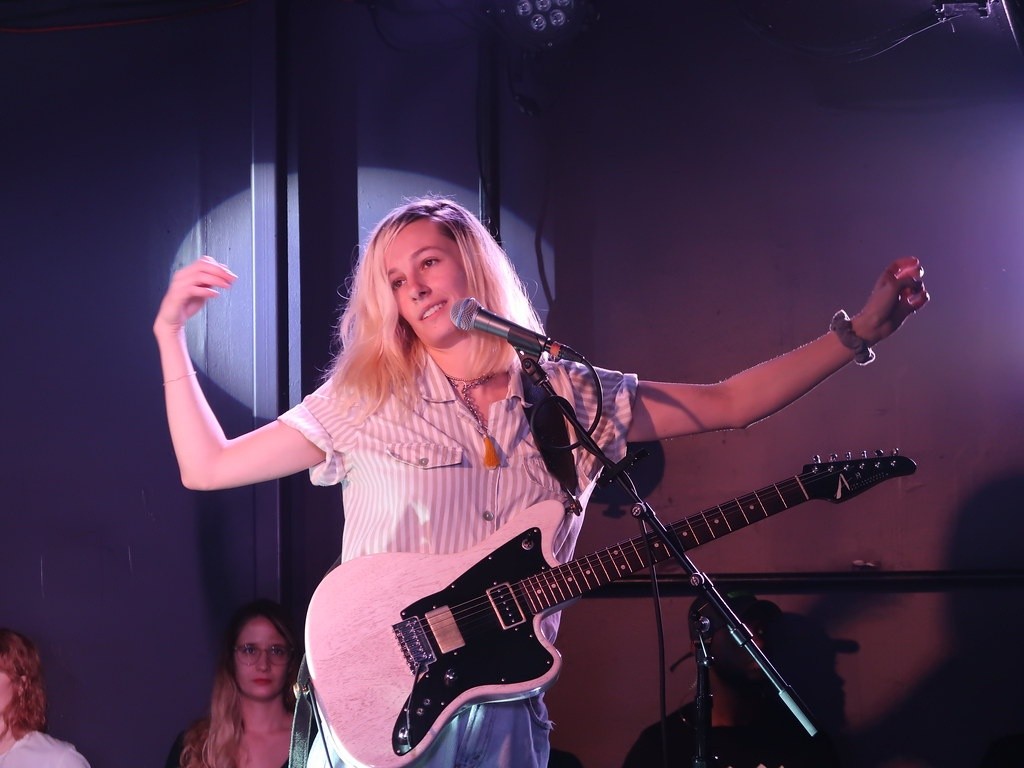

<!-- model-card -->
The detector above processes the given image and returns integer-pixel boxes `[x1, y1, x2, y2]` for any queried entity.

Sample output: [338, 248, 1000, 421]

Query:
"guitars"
[304, 449, 918, 768]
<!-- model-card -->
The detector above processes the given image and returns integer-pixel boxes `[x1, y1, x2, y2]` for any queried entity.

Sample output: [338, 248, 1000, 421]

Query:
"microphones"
[449, 296, 585, 364]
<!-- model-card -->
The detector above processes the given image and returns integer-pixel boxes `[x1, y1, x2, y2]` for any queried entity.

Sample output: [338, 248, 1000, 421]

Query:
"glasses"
[232, 643, 296, 665]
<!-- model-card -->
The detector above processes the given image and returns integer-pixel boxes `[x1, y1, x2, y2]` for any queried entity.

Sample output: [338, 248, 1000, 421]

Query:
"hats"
[687, 592, 757, 642]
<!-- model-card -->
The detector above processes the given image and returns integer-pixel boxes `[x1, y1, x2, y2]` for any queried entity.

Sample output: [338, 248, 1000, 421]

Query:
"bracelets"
[163, 370, 197, 386]
[829, 310, 875, 365]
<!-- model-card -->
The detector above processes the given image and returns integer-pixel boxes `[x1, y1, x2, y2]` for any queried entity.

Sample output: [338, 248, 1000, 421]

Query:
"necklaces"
[444, 372, 501, 470]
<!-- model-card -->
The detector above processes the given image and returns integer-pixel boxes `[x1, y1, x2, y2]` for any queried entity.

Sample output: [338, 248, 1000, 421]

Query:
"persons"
[0, 628, 91, 768]
[152, 199, 930, 768]
[165, 601, 318, 768]
[622, 591, 838, 768]
